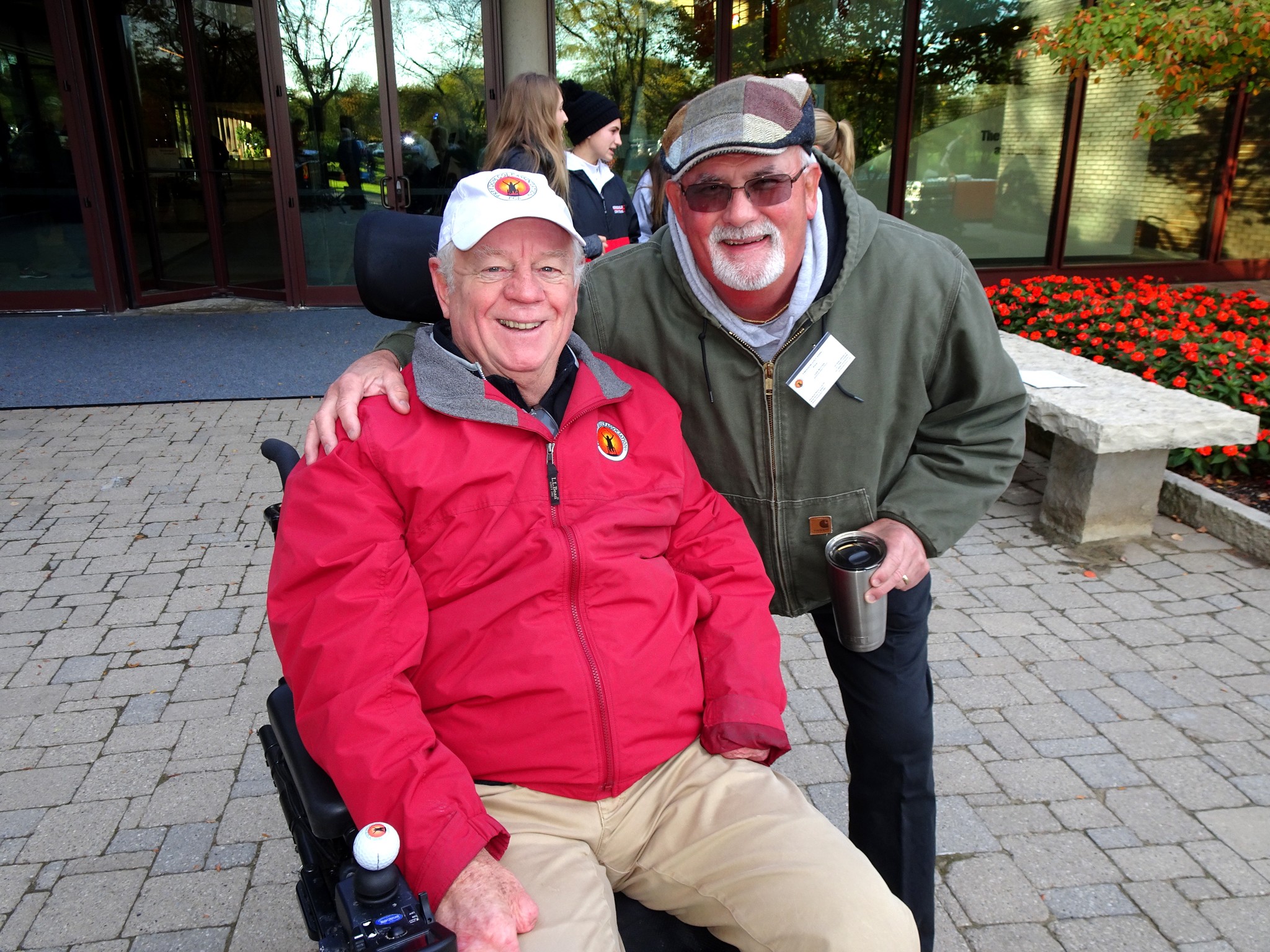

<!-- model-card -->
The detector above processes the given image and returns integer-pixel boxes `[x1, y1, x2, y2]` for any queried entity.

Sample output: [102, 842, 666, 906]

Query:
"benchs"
[996, 327, 1261, 545]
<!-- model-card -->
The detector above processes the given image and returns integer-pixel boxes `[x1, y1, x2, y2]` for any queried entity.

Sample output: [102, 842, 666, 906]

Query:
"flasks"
[824, 531, 887, 652]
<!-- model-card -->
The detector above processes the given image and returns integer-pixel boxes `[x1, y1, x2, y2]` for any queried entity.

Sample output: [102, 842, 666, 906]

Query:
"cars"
[355, 139, 384, 158]
[627, 136, 664, 161]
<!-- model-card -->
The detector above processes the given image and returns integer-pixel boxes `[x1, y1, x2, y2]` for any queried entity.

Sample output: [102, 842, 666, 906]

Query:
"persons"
[305, 76, 1030, 952]
[290, 115, 487, 215]
[480, 72, 856, 262]
[268, 169, 919, 952]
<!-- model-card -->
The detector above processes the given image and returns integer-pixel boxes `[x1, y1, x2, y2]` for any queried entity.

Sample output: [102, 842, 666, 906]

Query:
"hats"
[438, 169, 586, 252]
[559, 80, 622, 147]
[660, 75, 815, 182]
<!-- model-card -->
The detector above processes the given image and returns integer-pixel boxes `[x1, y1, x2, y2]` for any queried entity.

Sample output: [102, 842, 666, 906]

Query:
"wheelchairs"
[241, 437, 732, 950]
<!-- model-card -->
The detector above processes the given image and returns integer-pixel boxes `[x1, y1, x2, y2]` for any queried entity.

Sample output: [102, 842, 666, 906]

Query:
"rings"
[902, 574, 909, 586]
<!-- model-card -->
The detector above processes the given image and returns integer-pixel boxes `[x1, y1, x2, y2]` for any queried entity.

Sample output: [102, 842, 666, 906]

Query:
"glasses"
[679, 164, 809, 213]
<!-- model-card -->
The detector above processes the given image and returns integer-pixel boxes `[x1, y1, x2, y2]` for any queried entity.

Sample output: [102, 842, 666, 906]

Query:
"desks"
[947, 179, 996, 220]
